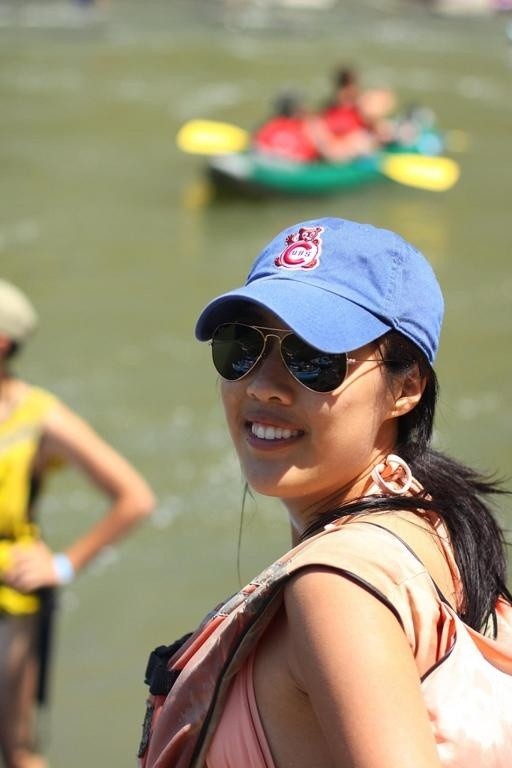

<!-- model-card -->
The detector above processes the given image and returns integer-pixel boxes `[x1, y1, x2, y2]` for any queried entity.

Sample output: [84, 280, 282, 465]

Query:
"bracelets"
[54, 554, 78, 582]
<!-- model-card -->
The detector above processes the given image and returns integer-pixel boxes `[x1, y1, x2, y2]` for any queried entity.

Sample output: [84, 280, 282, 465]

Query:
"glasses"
[207, 320, 410, 396]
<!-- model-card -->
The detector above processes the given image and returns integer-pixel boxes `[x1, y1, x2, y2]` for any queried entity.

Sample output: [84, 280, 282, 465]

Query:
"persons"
[2, 277, 156, 766]
[313, 61, 385, 156]
[137, 215, 512, 765]
[258, 96, 321, 161]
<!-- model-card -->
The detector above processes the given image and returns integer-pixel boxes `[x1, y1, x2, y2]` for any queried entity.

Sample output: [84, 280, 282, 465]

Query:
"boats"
[200, 127, 451, 202]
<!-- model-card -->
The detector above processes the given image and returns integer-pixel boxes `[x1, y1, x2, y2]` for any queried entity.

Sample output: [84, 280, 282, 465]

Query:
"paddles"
[176, 120, 255, 155]
[369, 150, 459, 193]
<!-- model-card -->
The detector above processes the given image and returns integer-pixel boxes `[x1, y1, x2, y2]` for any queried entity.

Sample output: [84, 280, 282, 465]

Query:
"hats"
[193, 215, 445, 367]
